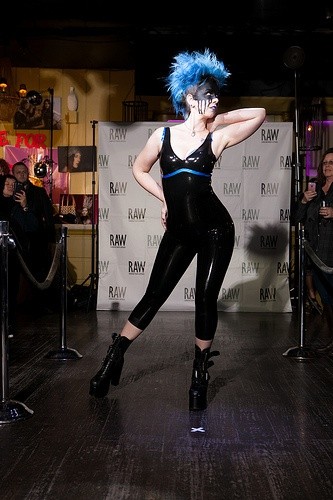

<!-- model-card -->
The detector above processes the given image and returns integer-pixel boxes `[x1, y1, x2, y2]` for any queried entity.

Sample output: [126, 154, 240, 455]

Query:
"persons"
[14, 99, 51, 129]
[0, 158, 63, 330]
[77, 207, 92, 225]
[39, 155, 65, 202]
[89, 49, 265, 409]
[287, 148, 333, 335]
[60, 147, 88, 172]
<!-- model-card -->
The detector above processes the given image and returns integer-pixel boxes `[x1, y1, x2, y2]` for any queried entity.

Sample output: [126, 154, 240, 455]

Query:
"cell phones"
[14, 182, 24, 199]
[308, 183, 316, 192]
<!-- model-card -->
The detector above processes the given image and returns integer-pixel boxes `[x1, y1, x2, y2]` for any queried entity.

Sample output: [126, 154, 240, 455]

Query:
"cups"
[319, 200, 328, 215]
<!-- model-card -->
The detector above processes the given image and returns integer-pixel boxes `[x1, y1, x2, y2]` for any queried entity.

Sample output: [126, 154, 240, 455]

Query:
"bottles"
[67, 86, 78, 111]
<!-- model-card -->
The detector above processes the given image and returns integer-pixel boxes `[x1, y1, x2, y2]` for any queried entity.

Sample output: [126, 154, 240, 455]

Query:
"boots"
[188, 343, 219, 411]
[90, 336, 134, 391]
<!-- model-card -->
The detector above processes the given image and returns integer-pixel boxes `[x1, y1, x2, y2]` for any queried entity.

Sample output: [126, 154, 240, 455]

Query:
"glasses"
[322, 160, 333, 166]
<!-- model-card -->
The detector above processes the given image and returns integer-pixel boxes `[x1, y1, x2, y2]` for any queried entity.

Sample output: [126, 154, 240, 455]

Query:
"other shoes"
[305, 300, 317, 314]
[312, 336, 333, 351]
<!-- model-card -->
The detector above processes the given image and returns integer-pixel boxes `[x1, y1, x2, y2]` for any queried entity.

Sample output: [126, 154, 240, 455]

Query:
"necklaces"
[184, 122, 205, 136]
